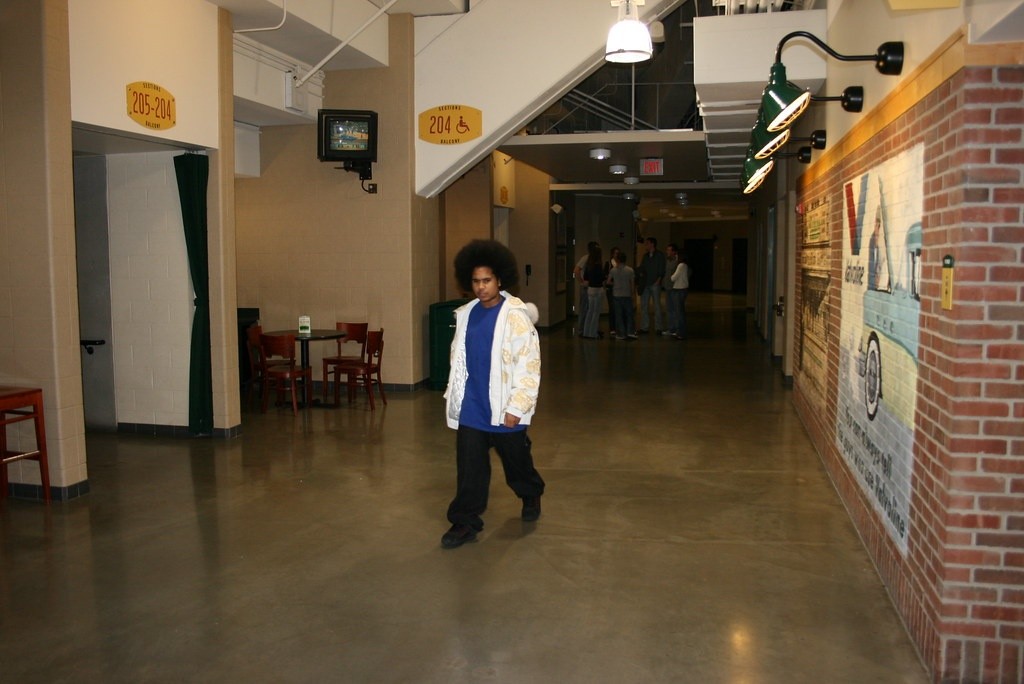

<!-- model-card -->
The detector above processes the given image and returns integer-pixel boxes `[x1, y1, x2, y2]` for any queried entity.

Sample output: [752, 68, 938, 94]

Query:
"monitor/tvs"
[318, 109, 378, 163]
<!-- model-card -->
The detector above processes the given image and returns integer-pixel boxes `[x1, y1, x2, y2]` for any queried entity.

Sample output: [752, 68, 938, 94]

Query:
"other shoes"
[627, 334, 638, 339]
[616, 335, 626, 339]
[677, 336, 686, 340]
[657, 330, 661, 335]
[638, 329, 647, 334]
[672, 333, 678, 337]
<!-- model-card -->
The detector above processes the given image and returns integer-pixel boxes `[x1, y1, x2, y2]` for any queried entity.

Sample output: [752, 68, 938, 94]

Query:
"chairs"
[333, 328, 387, 411]
[322, 321, 368, 400]
[246, 322, 312, 416]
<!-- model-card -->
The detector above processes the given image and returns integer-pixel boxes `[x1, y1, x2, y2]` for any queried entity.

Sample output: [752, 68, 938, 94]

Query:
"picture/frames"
[556, 201, 567, 249]
[554, 251, 567, 294]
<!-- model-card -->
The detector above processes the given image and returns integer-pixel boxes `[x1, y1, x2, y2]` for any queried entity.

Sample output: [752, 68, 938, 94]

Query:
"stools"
[0, 386, 51, 516]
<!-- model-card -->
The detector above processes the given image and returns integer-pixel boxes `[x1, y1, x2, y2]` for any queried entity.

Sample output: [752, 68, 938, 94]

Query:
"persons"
[573, 236, 691, 341]
[441, 239, 544, 549]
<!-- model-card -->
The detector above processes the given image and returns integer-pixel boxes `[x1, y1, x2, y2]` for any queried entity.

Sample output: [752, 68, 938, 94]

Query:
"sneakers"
[442, 524, 477, 546]
[521, 495, 541, 521]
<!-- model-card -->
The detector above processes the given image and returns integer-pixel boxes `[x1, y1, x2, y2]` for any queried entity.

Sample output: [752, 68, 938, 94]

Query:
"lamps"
[604, 0, 653, 63]
[760, 31, 904, 132]
[590, 148, 611, 160]
[624, 177, 639, 185]
[739, 79, 863, 195]
[609, 165, 627, 174]
[624, 192, 689, 218]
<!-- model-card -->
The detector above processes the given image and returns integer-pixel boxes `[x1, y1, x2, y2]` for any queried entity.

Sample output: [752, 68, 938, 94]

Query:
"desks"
[264, 328, 348, 411]
